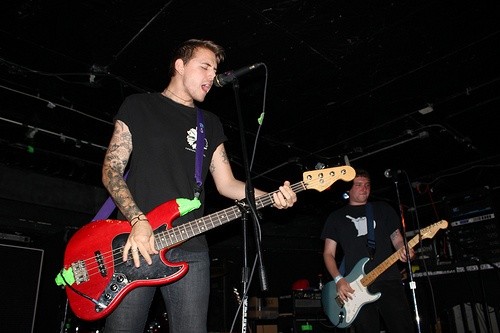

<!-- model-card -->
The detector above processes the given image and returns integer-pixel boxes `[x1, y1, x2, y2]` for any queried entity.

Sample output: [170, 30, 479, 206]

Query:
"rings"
[131, 249, 138, 252]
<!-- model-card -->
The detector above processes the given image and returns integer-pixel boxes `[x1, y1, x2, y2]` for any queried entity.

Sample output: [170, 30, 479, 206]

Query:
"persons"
[319, 171, 415, 333]
[102, 39, 296, 333]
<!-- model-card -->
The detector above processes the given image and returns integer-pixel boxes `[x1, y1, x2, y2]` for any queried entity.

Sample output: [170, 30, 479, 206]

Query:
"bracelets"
[334, 275, 343, 284]
[130, 213, 149, 228]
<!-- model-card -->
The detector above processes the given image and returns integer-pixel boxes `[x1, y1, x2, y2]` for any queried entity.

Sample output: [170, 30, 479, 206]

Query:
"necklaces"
[166, 88, 193, 102]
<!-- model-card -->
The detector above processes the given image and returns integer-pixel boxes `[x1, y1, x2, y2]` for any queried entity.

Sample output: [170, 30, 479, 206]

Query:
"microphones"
[384, 168, 406, 178]
[213, 64, 261, 88]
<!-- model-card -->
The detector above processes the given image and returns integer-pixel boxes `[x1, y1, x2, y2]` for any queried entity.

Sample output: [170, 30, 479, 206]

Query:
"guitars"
[56, 164, 356, 320]
[321, 219, 449, 328]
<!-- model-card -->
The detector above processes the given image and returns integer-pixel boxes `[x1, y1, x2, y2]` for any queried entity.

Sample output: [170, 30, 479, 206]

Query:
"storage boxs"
[278, 297, 324, 320]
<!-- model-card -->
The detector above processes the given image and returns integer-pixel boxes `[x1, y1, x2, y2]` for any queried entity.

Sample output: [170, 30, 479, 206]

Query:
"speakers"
[0, 240, 48, 333]
